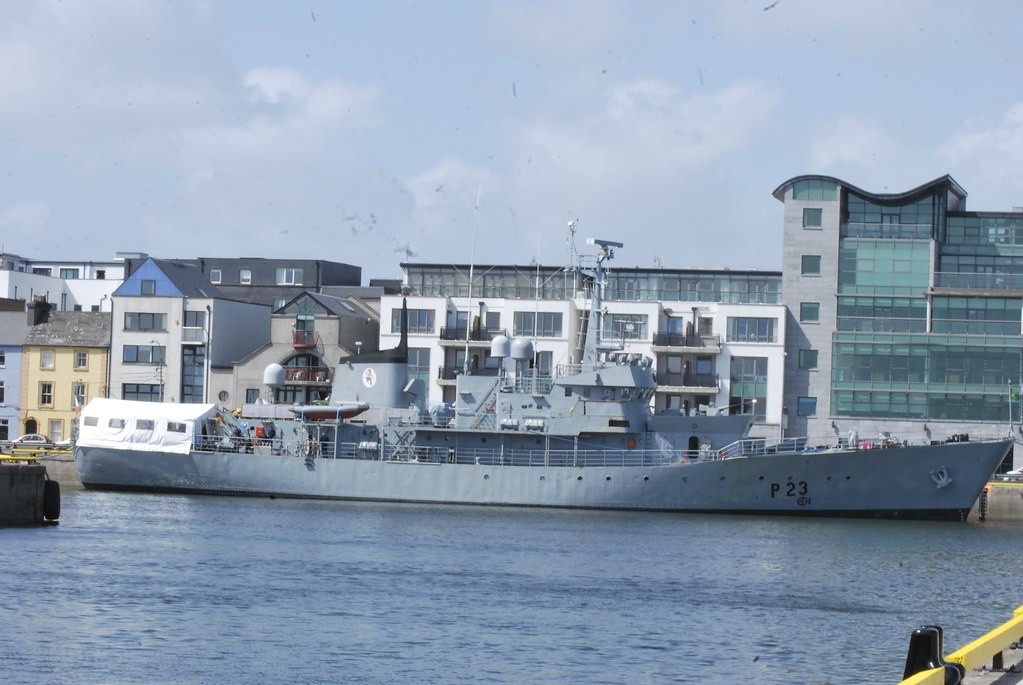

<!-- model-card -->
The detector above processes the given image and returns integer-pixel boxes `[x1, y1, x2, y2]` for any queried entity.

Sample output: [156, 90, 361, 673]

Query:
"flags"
[1011, 389, 1019, 401]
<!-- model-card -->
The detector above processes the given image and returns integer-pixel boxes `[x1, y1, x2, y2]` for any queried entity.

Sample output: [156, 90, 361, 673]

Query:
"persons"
[236, 429, 253, 450]
[304, 432, 316, 457]
[253, 426, 275, 446]
[320, 433, 330, 458]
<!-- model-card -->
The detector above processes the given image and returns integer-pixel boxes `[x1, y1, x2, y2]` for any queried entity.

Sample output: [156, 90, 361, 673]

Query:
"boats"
[0, 459, 63, 525]
[75, 236, 1017, 526]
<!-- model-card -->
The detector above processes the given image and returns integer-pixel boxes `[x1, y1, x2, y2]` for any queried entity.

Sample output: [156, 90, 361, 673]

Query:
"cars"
[2, 433, 52, 444]
[1003, 467, 1023, 481]
[53, 439, 71, 451]
